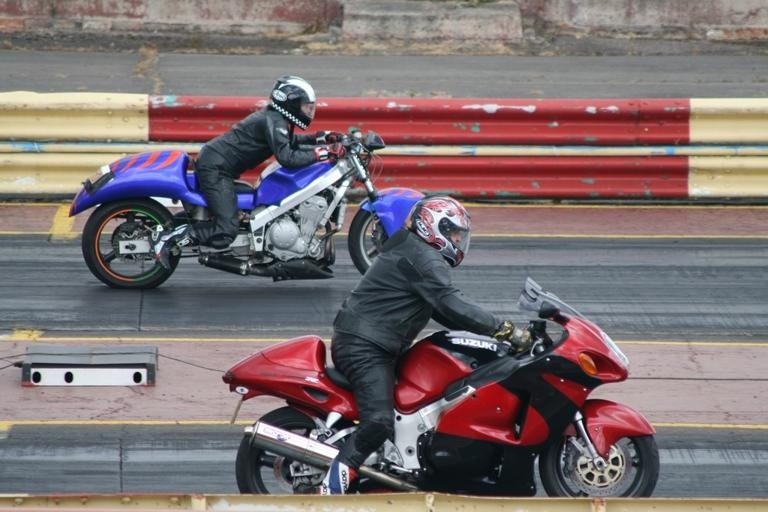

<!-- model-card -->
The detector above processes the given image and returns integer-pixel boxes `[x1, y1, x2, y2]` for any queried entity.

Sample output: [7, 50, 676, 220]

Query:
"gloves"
[315, 142, 347, 162]
[316, 130, 343, 144]
[494, 320, 533, 352]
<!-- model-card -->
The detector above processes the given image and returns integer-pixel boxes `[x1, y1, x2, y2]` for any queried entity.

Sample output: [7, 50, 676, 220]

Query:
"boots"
[320, 459, 359, 495]
[149, 221, 198, 273]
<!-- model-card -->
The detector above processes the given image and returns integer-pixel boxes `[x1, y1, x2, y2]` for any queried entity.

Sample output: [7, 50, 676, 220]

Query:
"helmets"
[405, 194, 472, 268]
[270, 75, 316, 131]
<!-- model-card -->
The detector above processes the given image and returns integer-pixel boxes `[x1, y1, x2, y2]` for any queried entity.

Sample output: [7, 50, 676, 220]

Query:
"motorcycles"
[221, 276, 660, 499]
[68, 126, 427, 292]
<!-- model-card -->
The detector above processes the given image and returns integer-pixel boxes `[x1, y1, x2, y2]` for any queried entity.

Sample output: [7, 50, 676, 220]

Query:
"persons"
[321, 195, 533, 496]
[148, 76, 345, 270]
